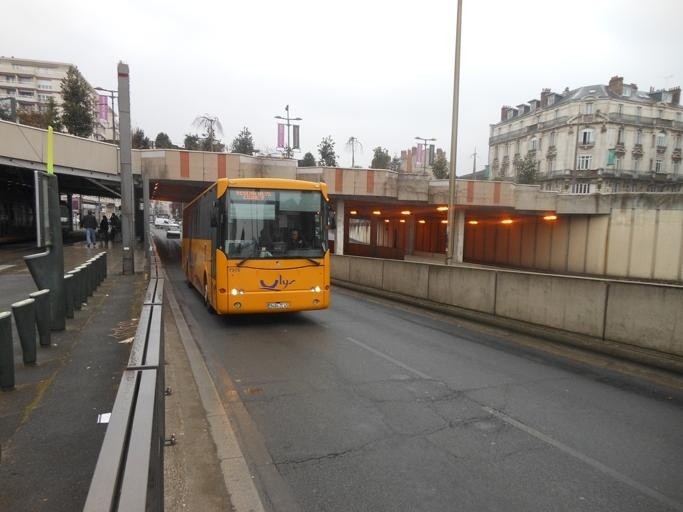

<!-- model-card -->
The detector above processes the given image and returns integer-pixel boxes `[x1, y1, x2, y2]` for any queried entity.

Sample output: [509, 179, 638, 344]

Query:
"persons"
[283, 229, 305, 249]
[97, 215, 108, 249]
[109, 213, 121, 242]
[78, 209, 96, 249]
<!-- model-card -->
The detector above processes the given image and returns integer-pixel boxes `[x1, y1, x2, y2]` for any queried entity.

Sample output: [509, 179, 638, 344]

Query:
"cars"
[148, 210, 183, 238]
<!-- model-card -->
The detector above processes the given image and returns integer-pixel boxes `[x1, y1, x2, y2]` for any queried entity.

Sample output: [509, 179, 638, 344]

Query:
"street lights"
[94, 87, 119, 144]
[275, 105, 303, 158]
[416, 137, 436, 174]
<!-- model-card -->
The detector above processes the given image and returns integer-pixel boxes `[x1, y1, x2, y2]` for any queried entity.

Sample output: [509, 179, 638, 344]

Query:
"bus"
[182, 177, 331, 315]
